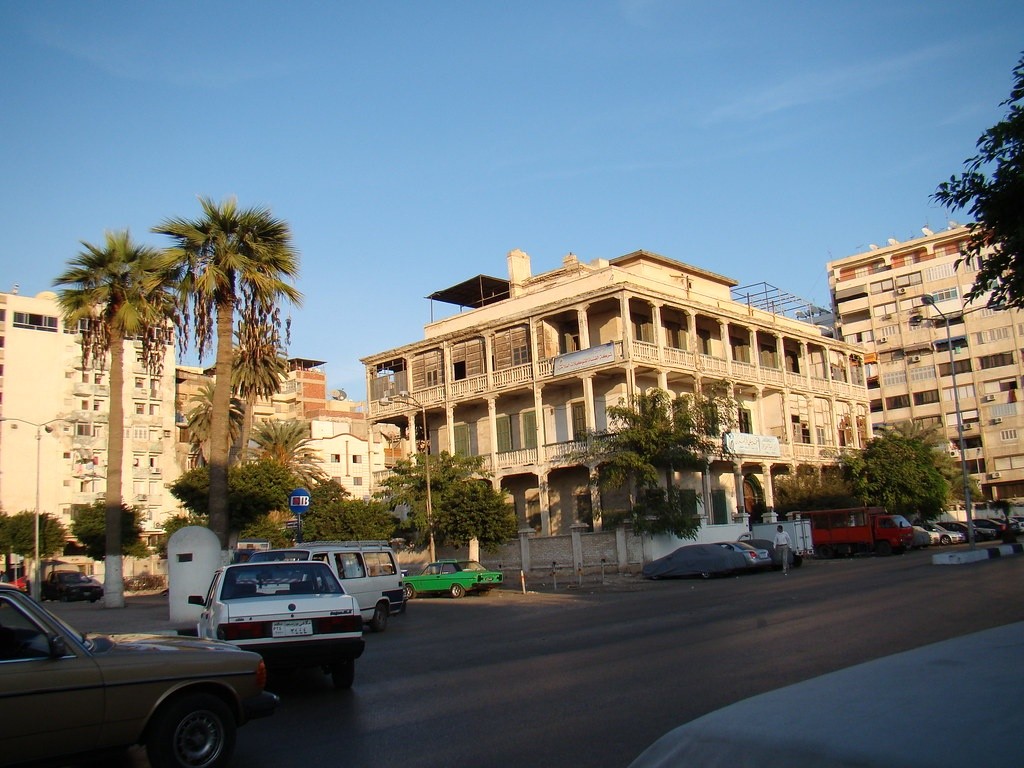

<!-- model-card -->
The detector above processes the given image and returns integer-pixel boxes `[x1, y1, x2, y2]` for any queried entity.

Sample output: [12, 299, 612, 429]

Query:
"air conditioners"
[137, 405, 142, 408]
[137, 379, 142, 384]
[138, 494, 148, 501]
[911, 355, 920, 362]
[897, 288, 907, 294]
[986, 394, 996, 401]
[151, 468, 161, 474]
[950, 450, 958, 457]
[992, 472, 1001, 478]
[880, 337, 887, 342]
[994, 417, 1002, 423]
[852, 355, 859, 360]
[165, 432, 171, 437]
[964, 423, 972, 430]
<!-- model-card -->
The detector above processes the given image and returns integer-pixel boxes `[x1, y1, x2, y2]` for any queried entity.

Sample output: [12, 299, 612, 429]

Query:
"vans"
[236, 540, 406, 633]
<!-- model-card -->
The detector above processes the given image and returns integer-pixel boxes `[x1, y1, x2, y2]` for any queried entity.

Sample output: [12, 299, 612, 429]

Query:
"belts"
[778, 544, 788, 546]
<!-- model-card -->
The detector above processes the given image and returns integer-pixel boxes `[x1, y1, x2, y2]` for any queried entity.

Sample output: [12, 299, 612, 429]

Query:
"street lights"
[906, 293, 1006, 552]
[0, 412, 83, 605]
[378, 391, 437, 575]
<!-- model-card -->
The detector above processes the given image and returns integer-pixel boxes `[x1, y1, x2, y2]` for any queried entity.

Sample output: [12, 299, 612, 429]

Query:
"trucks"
[783, 506, 914, 558]
[737, 517, 815, 567]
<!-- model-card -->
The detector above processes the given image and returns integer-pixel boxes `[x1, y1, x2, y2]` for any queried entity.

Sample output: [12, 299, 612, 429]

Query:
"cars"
[910, 525, 941, 549]
[935, 520, 999, 542]
[402, 559, 504, 599]
[641, 543, 746, 579]
[187, 561, 364, 694]
[44, 570, 105, 603]
[916, 521, 963, 546]
[0, 590, 281, 768]
[970, 519, 1007, 539]
[713, 541, 772, 573]
[992, 515, 1024, 537]
[7, 575, 28, 591]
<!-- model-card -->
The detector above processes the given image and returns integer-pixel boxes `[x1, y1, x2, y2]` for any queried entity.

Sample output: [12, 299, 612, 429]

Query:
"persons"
[774, 525, 791, 575]
